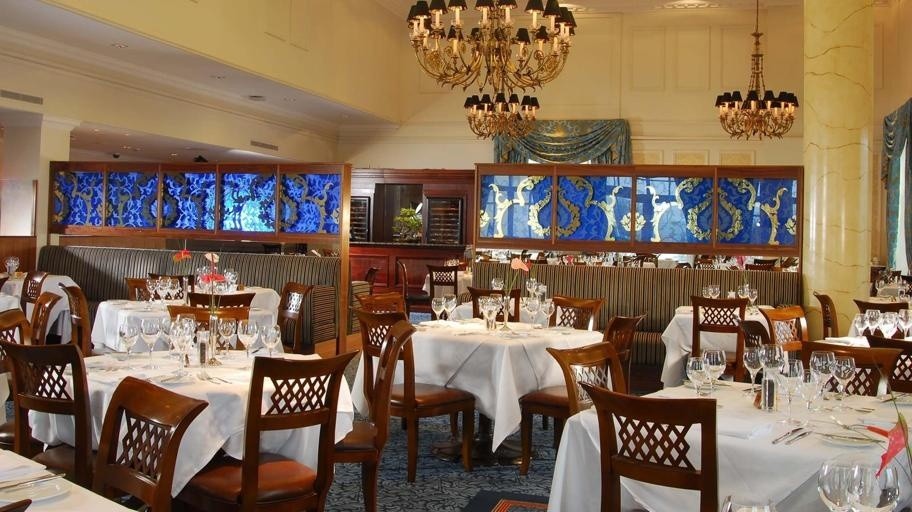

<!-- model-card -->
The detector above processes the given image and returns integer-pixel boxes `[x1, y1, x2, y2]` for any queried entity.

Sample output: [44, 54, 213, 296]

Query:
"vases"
[500, 295, 512, 332]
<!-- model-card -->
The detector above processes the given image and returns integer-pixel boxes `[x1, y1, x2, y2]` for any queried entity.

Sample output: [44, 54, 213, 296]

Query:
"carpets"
[457, 487, 549, 512]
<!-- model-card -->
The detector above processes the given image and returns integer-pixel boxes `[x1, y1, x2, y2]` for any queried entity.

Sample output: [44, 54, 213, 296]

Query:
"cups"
[196, 330, 210, 366]
[831, 358, 855, 399]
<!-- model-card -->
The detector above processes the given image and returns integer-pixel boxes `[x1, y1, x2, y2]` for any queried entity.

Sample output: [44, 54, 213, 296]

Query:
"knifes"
[880, 390, 905, 405]
[785, 431, 811, 445]
[816, 430, 884, 445]
[772, 425, 803, 444]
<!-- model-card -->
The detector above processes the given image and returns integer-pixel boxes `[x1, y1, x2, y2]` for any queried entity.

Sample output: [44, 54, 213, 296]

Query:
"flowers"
[848, 379, 912, 483]
[504, 256, 530, 297]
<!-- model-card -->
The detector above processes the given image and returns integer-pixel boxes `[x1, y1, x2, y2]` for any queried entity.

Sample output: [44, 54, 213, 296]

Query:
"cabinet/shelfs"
[349, 245, 466, 312]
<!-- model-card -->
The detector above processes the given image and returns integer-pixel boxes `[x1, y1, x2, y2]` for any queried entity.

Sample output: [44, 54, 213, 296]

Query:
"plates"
[813, 420, 885, 449]
[0, 476, 68, 505]
[151, 372, 196, 385]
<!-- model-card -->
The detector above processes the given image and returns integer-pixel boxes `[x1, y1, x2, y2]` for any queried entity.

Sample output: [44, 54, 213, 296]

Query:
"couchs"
[349, 262, 371, 334]
[37, 244, 342, 354]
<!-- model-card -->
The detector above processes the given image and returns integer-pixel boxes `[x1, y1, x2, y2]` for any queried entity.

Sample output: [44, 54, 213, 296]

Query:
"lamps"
[716, 1, 801, 139]
[407, 0, 577, 138]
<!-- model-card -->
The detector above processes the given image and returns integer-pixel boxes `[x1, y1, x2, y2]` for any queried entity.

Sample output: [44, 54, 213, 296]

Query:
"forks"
[198, 368, 231, 386]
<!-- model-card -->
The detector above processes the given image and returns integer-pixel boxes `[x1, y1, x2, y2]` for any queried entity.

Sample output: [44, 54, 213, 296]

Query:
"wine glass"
[874, 280, 884, 299]
[757, 344, 784, 387]
[117, 323, 140, 361]
[897, 308, 912, 341]
[491, 277, 503, 292]
[779, 360, 804, 428]
[707, 284, 721, 300]
[442, 294, 456, 322]
[702, 287, 710, 299]
[810, 351, 834, 409]
[748, 289, 757, 319]
[534, 285, 545, 304]
[524, 297, 541, 330]
[142, 318, 162, 372]
[590, 255, 597, 267]
[169, 321, 192, 377]
[702, 349, 727, 393]
[431, 297, 446, 325]
[4, 255, 20, 280]
[224, 267, 238, 292]
[182, 317, 196, 365]
[879, 312, 899, 339]
[685, 356, 711, 399]
[742, 347, 761, 394]
[817, 460, 862, 511]
[717, 496, 777, 511]
[220, 316, 237, 356]
[237, 319, 258, 363]
[846, 466, 899, 512]
[168, 278, 179, 303]
[162, 318, 179, 362]
[525, 278, 537, 299]
[260, 324, 282, 357]
[156, 280, 169, 306]
[197, 264, 210, 291]
[583, 257, 590, 266]
[479, 294, 503, 333]
[737, 284, 750, 298]
[897, 288, 911, 303]
[865, 310, 882, 336]
[542, 301, 555, 330]
[145, 279, 158, 304]
[159, 276, 172, 290]
[854, 315, 869, 335]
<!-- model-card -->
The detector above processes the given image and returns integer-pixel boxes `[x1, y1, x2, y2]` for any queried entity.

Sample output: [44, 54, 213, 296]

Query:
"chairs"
[468, 286, 520, 322]
[0, 309, 44, 453]
[0, 499, 32, 512]
[21, 272, 49, 314]
[278, 281, 310, 354]
[814, 290, 838, 339]
[803, 340, 903, 397]
[350, 307, 475, 482]
[148, 272, 195, 300]
[691, 296, 748, 382]
[760, 305, 808, 352]
[366, 267, 380, 294]
[355, 291, 404, 313]
[126, 277, 161, 300]
[518, 315, 646, 476]
[189, 293, 256, 308]
[58, 283, 92, 357]
[29, 293, 63, 344]
[0, 339, 100, 489]
[854, 299, 910, 338]
[397, 260, 437, 320]
[0, 277, 9, 291]
[580, 381, 718, 512]
[545, 342, 628, 414]
[170, 349, 359, 511]
[334, 320, 417, 511]
[870, 266, 912, 298]
[867, 334, 912, 393]
[427, 265, 457, 320]
[167, 305, 250, 351]
[92, 375, 209, 512]
[543, 295, 605, 429]
